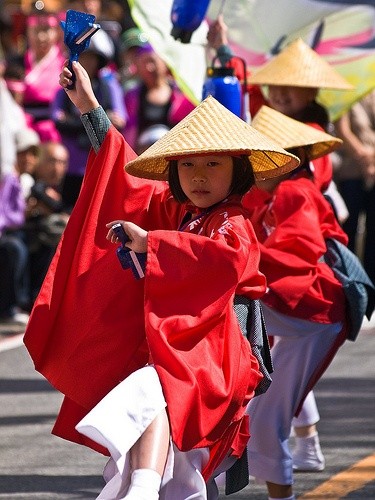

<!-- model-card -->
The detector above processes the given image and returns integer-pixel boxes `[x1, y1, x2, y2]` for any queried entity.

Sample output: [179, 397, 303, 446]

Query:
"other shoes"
[0, 312, 30, 333]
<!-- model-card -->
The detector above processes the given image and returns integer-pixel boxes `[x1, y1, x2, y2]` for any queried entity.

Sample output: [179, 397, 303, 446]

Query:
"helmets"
[61, 28, 114, 68]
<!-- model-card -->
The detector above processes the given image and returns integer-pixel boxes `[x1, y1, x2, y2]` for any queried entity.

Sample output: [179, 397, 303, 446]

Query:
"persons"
[1, 0, 374, 351]
[239, 105, 375, 500]
[22, 60, 303, 500]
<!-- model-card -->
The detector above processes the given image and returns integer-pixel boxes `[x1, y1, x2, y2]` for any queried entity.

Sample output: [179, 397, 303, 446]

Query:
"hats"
[240, 37, 354, 90]
[250, 104, 342, 160]
[13, 127, 39, 152]
[118, 28, 151, 52]
[124, 92, 300, 183]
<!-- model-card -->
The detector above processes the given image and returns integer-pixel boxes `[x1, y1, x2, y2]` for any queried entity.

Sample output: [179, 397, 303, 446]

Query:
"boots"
[289, 432, 326, 471]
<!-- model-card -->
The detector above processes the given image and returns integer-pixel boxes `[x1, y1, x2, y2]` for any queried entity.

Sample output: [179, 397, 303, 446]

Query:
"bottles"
[202, 68, 242, 120]
